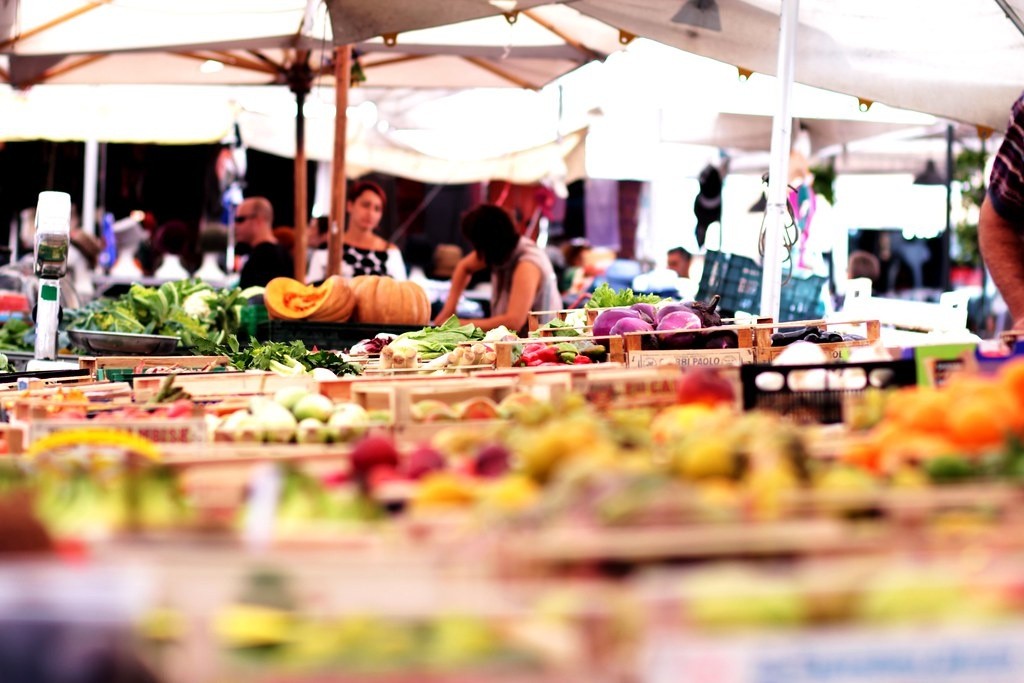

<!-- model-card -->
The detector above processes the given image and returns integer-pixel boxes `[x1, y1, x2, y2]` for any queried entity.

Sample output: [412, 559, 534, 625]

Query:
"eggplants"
[591, 294, 867, 351]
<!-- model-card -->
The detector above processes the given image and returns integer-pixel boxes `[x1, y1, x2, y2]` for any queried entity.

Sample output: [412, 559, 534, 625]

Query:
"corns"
[214, 386, 369, 447]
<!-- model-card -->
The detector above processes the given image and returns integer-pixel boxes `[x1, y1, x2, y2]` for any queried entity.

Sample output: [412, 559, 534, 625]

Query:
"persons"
[306, 217, 328, 270]
[432, 204, 562, 337]
[977, 91, 1024, 335]
[835, 252, 879, 307]
[306, 181, 407, 285]
[666, 247, 691, 279]
[235, 198, 294, 290]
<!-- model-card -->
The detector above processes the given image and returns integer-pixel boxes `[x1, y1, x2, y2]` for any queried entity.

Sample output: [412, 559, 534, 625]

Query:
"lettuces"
[585, 283, 671, 309]
[381, 313, 580, 360]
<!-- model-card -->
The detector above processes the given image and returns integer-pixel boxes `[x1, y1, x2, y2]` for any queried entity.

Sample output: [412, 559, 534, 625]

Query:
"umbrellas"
[0, 0, 629, 285]
[333, 0, 1024, 332]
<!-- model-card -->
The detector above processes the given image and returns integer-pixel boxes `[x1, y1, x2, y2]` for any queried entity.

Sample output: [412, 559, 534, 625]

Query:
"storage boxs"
[694, 249, 830, 322]
[0, 320, 1024, 490]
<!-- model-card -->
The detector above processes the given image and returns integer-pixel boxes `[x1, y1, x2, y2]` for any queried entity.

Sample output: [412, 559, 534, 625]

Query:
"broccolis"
[82, 280, 238, 350]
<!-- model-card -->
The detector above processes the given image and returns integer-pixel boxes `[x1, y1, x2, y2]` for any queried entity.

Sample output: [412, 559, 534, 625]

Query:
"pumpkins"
[263, 274, 354, 323]
[343, 274, 432, 325]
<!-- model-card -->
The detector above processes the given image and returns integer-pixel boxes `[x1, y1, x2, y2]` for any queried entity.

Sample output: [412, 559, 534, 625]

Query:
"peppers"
[515, 343, 607, 367]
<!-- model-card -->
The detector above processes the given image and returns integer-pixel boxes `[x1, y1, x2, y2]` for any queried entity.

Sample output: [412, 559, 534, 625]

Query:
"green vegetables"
[212, 333, 365, 382]
